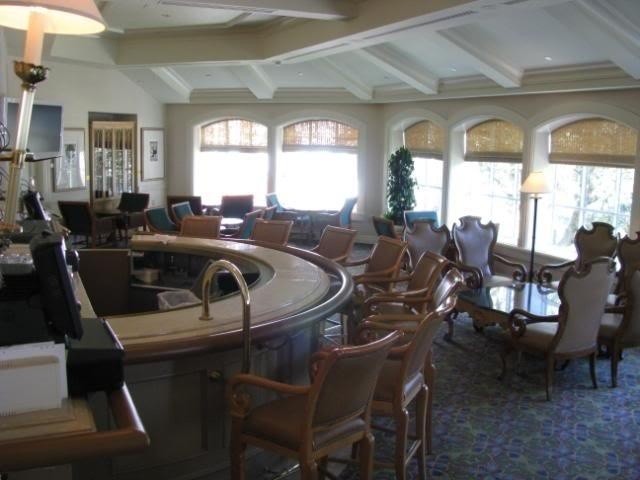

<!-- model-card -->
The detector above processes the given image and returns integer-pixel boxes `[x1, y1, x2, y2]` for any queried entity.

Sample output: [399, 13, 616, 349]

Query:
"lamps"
[0, 0, 111, 233]
[520, 171, 553, 283]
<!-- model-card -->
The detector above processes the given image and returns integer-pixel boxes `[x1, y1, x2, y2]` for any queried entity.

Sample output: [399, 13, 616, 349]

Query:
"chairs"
[146, 194, 358, 244]
[371, 210, 639, 399]
[57, 198, 122, 249]
[117, 187, 151, 242]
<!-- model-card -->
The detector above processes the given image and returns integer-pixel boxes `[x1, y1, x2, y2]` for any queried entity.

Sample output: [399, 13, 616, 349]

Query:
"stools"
[145, 216, 462, 476]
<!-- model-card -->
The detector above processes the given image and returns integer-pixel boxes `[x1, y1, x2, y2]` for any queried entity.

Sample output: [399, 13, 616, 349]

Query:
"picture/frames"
[51, 127, 87, 194]
[138, 126, 167, 184]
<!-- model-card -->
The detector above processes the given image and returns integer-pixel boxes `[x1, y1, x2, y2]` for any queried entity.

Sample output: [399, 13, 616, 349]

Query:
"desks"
[93, 208, 125, 243]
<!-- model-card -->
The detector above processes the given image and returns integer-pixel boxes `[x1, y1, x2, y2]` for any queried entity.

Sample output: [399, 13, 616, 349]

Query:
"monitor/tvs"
[0, 95, 64, 160]
[29, 230, 84, 342]
[23, 191, 52, 222]
